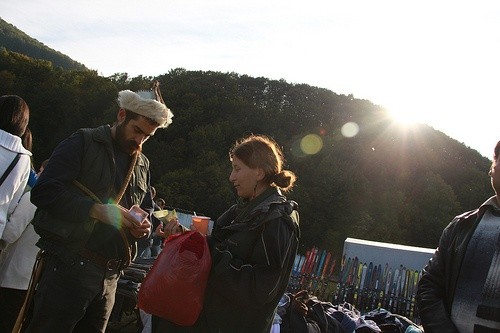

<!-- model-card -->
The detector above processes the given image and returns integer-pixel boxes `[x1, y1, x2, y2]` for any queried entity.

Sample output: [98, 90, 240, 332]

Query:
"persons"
[0, 95, 165, 333]
[27, 80, 174, 333]
[416, 140, 500, 333]
[154, 137, 301, 333]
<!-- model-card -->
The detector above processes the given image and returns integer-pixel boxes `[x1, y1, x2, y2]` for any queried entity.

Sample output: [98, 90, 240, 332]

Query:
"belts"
[81, 247, 122, 272]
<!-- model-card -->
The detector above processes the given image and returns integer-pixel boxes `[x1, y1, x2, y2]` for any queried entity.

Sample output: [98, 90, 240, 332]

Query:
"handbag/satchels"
[138, 230, 211, 326]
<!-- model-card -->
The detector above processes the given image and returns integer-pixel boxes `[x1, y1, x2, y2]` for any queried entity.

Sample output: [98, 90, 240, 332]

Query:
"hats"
[118, 81, 173, 126]
[355, 320, 381, 331]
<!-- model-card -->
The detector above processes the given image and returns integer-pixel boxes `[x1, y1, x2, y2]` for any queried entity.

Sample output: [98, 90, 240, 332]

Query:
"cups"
[192, 216, 211, 237]
